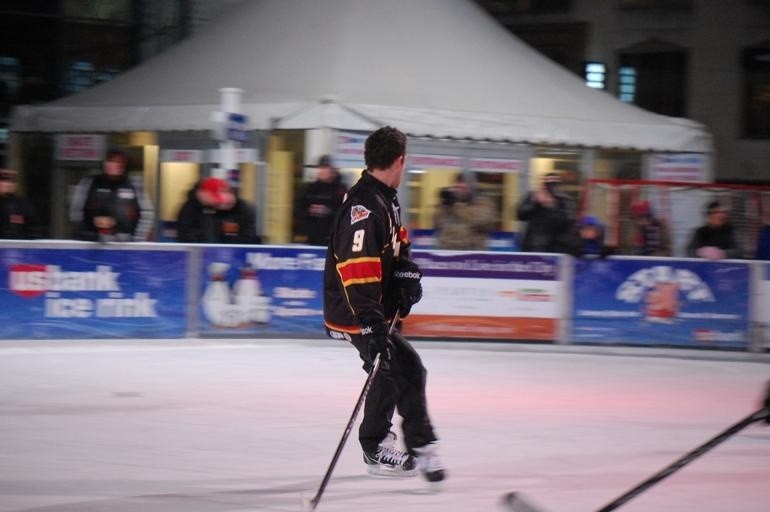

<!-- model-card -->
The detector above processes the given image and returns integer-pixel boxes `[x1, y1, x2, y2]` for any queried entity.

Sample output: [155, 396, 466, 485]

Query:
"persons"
[291, 157, 349, 247]
[514, 186, 574, 254]
[631, 201, 673, 254]
[82, 152, 140, 244]
[564, 216, 610, 261]
[755, 224, 770, 261]
[173, 178, 232, 244]
[685, 201, 741, 260]
[761, 385, 770, 422]
[322, 127, 445, 481]
[0, 168, 26, 238]
[219, 189, 261, 245]
[433, 178, 494, 250]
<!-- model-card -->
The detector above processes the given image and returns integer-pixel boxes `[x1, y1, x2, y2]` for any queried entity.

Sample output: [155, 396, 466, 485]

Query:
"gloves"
[360, 310, 396, 371]
[392, 260, 423, 318]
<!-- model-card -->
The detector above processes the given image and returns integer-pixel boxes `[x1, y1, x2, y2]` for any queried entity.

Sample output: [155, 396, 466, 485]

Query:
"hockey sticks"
[509, 407, 768, 511]
[302, 310, 401, 511]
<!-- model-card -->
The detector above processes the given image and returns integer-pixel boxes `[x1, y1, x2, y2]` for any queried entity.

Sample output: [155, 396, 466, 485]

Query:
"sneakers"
[363, 445, 416, 470]
[412, 441, 444, 481]
